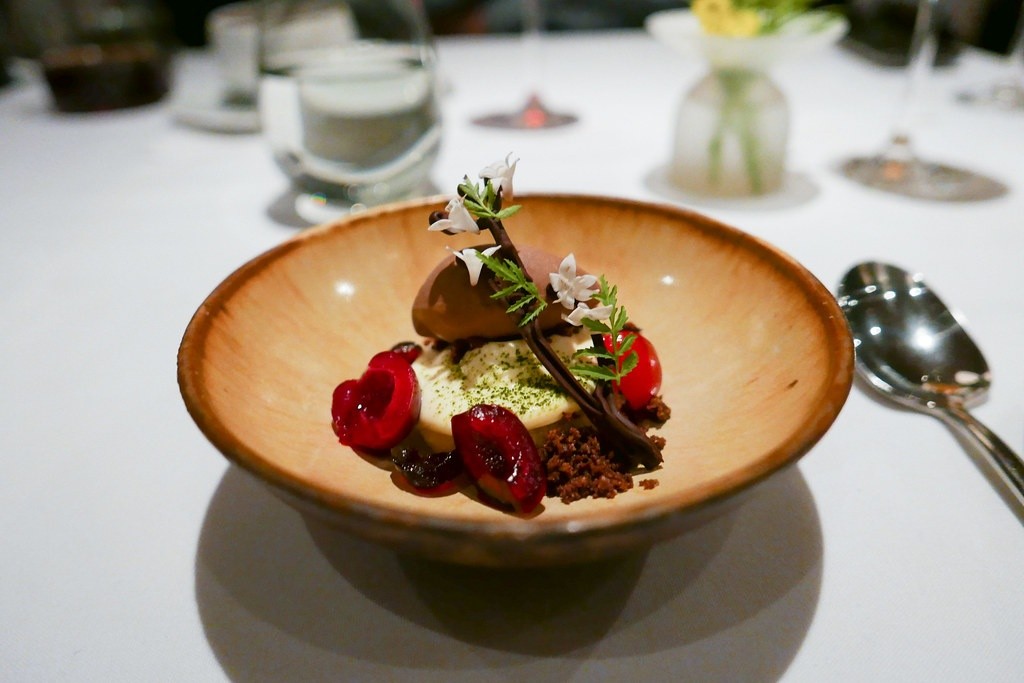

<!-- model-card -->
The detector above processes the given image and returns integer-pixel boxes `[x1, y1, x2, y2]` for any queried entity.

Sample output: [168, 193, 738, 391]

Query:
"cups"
[256, 0, 444, 224]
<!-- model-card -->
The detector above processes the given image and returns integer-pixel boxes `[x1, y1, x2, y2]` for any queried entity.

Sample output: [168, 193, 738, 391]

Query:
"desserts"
[330, 151, 671, 507]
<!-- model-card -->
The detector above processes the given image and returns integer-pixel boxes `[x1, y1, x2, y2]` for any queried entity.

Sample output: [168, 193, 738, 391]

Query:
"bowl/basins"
[175, 190, 854, 573]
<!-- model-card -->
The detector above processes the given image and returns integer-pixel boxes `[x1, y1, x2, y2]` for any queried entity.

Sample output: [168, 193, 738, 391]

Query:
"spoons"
[837, 261, 1024, 507]
